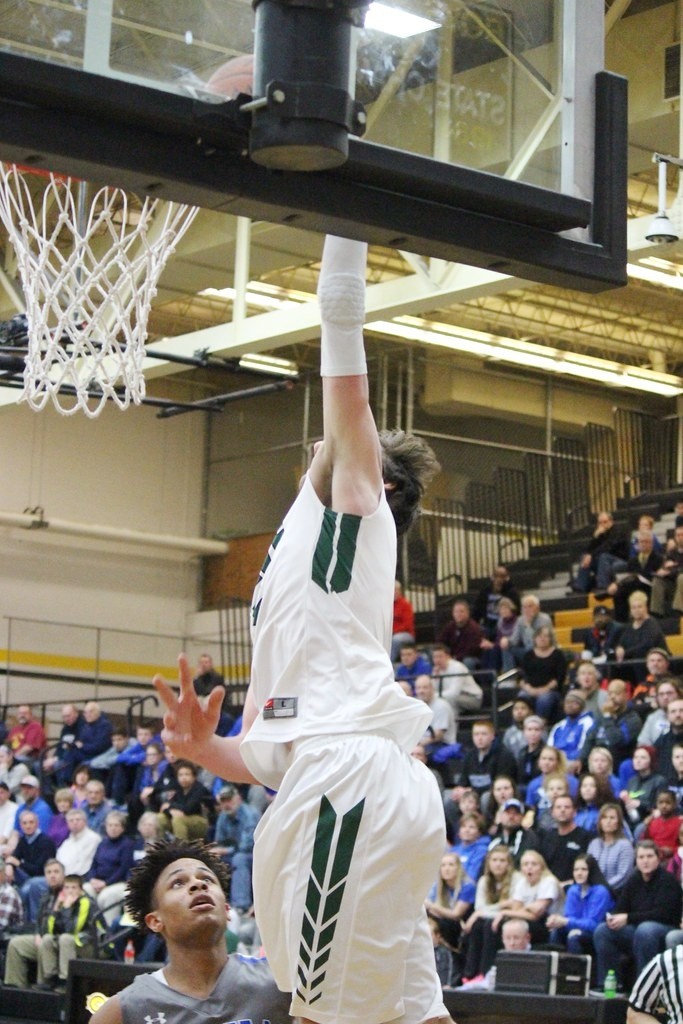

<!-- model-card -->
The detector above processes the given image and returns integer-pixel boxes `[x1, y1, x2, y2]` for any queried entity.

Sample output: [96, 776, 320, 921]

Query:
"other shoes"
[31, 982, 58, 992]
[54, 985, 67, 993]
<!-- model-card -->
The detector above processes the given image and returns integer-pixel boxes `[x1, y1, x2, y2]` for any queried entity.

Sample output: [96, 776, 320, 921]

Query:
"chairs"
[438, 487, 683, 727]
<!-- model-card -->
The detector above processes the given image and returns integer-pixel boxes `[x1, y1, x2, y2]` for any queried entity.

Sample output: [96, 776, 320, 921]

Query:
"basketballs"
[203, 54, 253, 99]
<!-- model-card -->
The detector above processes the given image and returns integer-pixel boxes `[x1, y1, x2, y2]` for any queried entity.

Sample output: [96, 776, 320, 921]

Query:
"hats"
[220, 785, 237, 801]
[504, 798, 524, 813]
[594, 606, 608, 615]
[568, 691, 585, 708]
[21, 774, 39, 788]
[637, 744, 655, 764]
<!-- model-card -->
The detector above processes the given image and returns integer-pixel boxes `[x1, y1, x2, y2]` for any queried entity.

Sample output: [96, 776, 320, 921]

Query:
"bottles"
[123, 940, 135, 965]
[604, 969, 616, 999]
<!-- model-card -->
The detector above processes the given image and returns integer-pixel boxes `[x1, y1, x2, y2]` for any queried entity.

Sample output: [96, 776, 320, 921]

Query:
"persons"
[626, 944, 683, 1024]
[390, 502, 683, 995]
[0, 654, 278, 995]
[87, 836, 297, 1024]
[152, 230, 458, 1024]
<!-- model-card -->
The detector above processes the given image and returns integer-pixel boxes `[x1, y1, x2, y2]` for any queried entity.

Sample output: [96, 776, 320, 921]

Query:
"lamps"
[645, 153, 683, 243]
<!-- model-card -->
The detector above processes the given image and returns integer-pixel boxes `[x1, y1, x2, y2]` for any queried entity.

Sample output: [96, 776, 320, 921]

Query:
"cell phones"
[606, 912, 613, 923]
